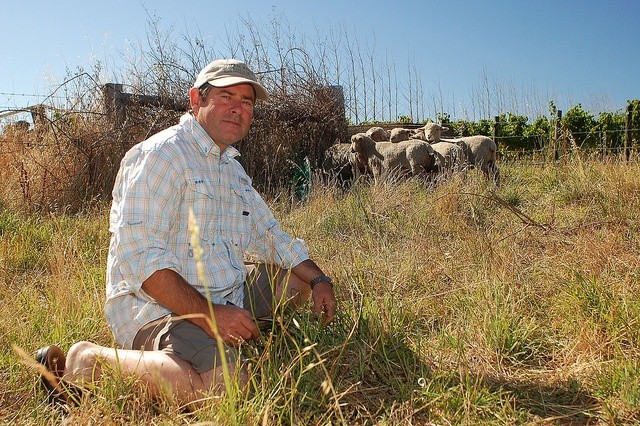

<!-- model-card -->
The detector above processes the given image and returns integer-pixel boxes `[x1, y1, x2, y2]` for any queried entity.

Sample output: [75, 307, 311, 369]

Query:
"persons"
[35, 59, 337, 413]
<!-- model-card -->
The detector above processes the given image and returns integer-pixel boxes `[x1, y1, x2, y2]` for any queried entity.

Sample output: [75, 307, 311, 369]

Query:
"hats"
[192, 58, 269, 101]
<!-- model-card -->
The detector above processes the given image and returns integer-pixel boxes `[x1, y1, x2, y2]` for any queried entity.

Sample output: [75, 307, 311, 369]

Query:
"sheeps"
[322, 125, 388, 181]
[388, 127, 467, 175]
[419, 121, 501, 186]
[349, 131, 434, 188]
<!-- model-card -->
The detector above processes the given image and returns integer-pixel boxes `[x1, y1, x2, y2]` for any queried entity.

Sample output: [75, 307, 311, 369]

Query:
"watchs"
[310, 276, 334, 289]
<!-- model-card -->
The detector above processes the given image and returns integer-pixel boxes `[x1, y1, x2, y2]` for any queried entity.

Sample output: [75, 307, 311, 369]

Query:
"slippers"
[33, 344, 94, 417]
